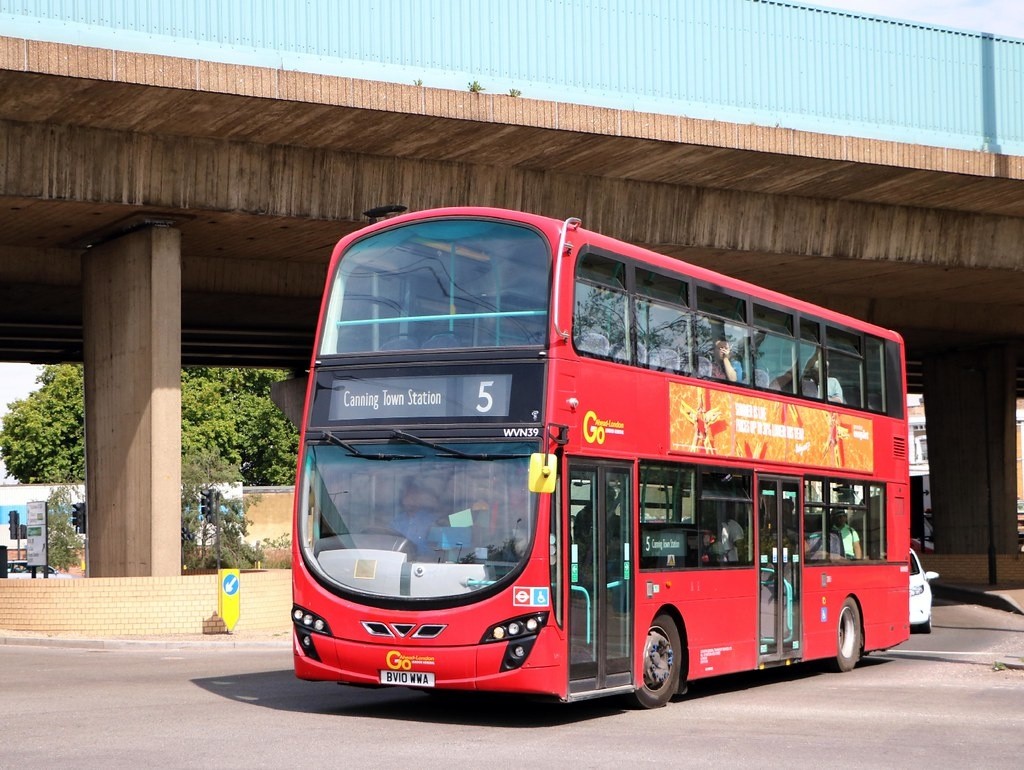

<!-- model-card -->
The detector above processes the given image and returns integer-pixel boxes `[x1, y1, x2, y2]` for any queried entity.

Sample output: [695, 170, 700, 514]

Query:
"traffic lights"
[71, 503, 87, 534]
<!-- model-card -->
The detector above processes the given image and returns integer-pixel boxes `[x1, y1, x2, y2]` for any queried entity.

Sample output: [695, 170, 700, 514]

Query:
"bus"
[287, 205, 911, 709]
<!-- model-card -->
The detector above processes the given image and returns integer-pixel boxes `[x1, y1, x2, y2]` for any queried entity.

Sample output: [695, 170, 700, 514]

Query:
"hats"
[830, 508, 847, 518]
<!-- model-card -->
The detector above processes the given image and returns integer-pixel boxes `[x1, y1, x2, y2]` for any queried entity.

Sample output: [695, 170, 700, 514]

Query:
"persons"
[711, 336, 743, 382]
[391, 482, 439, 559]
[570, 482, 865, 654]
[814, 358, 843, 404]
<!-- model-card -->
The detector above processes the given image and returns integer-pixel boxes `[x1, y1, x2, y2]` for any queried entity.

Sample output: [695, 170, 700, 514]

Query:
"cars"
[908, 548, 940, 633]
[4, 562, 79, 579]
